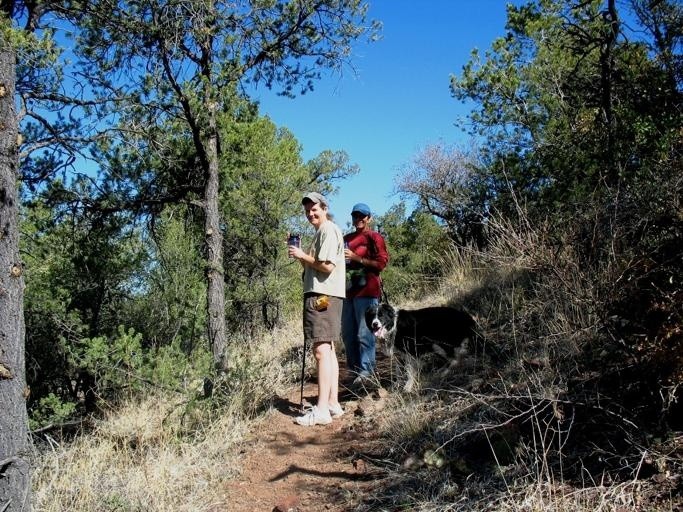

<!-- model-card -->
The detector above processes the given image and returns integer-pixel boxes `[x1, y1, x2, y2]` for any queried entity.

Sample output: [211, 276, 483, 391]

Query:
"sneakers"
[295, 404, 344, 426]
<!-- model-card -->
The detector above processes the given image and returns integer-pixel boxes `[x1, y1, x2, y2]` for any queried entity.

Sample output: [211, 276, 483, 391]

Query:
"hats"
[351, 203, 371, 217]
[302, 192, 328, 207]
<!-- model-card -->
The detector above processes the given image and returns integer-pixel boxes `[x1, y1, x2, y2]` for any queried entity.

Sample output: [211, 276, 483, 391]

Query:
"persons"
[283, 191, 346, 427]
[343, 202, 391, 385]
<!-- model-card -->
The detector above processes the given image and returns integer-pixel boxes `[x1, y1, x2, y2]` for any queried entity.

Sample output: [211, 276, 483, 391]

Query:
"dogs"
[364, 303, 478, 394]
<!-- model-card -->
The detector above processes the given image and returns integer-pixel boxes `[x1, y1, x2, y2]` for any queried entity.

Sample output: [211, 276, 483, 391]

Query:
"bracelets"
[360, 255, 363, 264]
[366, 256, 369, 265]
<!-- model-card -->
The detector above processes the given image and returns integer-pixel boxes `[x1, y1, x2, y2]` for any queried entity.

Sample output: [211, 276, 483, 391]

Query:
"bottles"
[288, 234, 299, 258]
[345, 241, 350, 263]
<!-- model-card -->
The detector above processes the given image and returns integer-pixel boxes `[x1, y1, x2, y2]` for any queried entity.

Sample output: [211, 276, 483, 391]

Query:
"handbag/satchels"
[345, 268, 367, 297]
